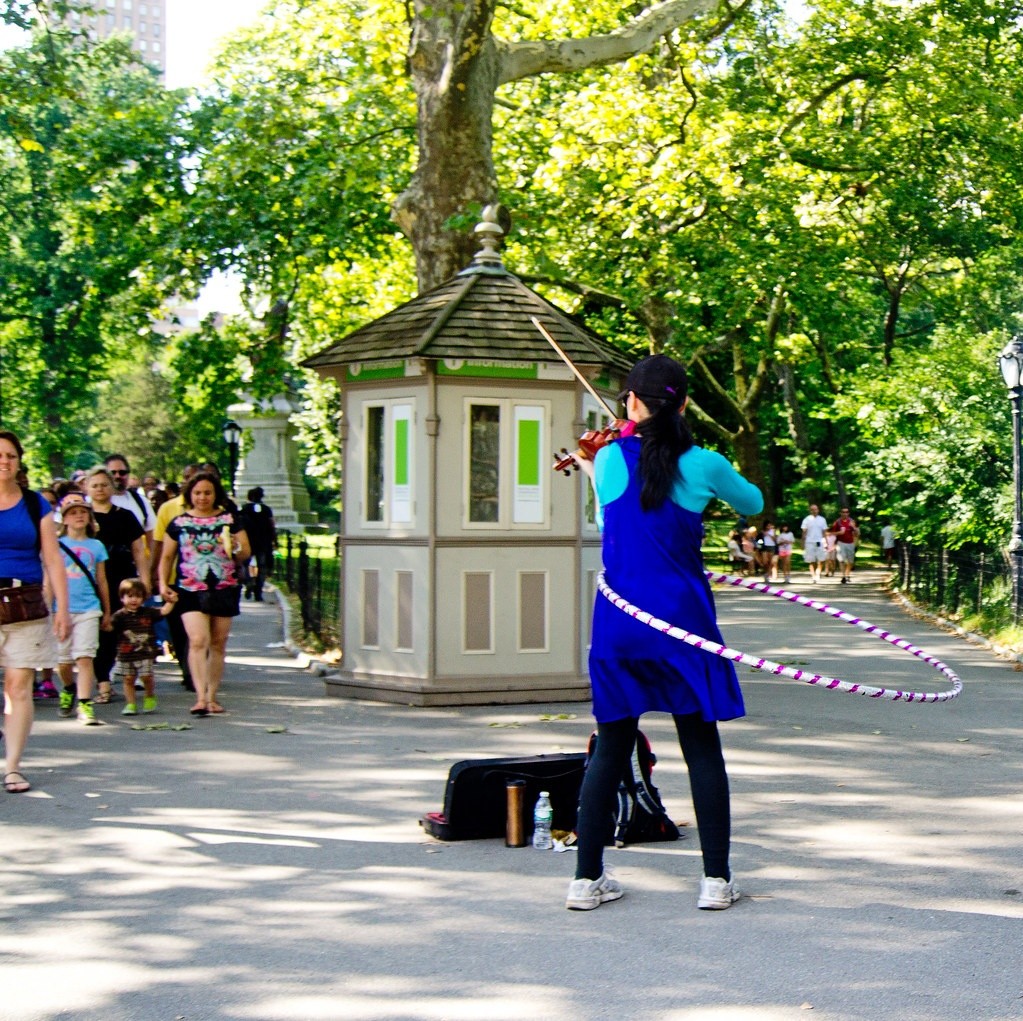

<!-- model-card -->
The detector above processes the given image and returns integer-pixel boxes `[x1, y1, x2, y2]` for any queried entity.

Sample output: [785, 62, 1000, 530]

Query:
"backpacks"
[563, 726, 684, 849]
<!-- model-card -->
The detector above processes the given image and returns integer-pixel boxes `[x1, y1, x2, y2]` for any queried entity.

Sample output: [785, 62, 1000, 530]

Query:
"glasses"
[49, 500, 58, 505]
[110, 469, 130, 476]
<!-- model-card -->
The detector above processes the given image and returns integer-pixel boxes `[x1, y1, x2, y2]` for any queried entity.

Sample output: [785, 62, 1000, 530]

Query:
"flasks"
[506, 779, 527, 847]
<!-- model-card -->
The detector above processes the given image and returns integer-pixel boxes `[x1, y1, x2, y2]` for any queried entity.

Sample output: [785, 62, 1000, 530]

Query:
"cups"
[840, 527, 846, 531]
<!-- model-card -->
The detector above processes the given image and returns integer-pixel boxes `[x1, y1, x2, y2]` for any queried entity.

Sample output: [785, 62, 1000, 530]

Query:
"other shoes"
[245, 590, 252, 599]
[846, 578, 851, 583]
[93, 685, 119, 704]
[254, 593, 263, 602]
[142, 695, 159, 713]
[32, 678, 59, 700]
[191, 700, 209, 716]
[783, 577, 791, 583]
[765, 578, 770, 582]
[206, 701, 225, 713]
[841, 577, 846, 584]
[121, 703, 140, 715]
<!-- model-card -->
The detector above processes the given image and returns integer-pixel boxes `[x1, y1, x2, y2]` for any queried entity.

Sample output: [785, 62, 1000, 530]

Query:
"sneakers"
[57, 683, 77, 717]
[566, 863, 624, 910]
[76, 700, 98, 725]
[697, 869, 741, 909]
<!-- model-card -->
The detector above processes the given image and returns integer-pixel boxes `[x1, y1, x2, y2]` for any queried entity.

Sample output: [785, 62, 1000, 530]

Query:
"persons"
[17, 452, 279, 718]
[565, 354, 764, 910]
[41, 493, 110, 724]
[0, 432, 72, 794]
[727, 504, 861, 584]
[879, 522, 895, 569]
[104, 578, 177, 715]
[156, 471, 250, 715]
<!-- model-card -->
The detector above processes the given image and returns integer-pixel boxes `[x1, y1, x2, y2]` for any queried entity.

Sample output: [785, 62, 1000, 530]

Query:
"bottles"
[532, 791, 553, 849]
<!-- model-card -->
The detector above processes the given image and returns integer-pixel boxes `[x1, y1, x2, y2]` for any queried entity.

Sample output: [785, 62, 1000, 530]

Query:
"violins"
[551, 418, 640, 477]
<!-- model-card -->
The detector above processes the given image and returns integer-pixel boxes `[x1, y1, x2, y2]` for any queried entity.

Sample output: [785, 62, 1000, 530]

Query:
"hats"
[616, 353, 688, 400]
[59, 494, 92, 514]
[71, 470, 87, 483]
[248, 487, 264, 502]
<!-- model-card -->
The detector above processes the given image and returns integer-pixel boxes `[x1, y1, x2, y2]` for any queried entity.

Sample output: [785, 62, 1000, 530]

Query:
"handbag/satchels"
[248, 556, 258, 577]
[0, 581, 50, 625]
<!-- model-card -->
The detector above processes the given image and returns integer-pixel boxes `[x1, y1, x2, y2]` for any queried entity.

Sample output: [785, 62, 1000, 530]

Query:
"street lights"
[994, 334, 1023, 629]
[220, 419, 243, 496]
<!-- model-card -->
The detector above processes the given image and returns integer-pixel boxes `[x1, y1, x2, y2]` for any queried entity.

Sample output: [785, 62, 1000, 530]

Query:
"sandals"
[3, 772, 32, 794]
